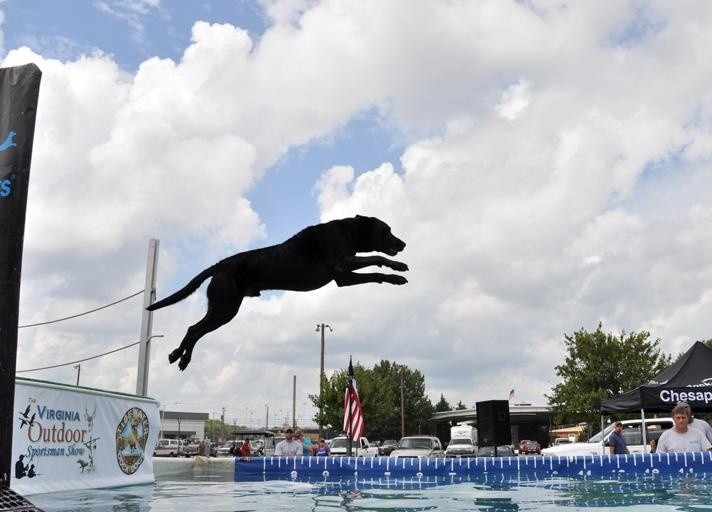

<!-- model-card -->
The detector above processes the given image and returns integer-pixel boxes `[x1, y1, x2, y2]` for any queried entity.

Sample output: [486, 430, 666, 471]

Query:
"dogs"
[143, 214, 409, 371]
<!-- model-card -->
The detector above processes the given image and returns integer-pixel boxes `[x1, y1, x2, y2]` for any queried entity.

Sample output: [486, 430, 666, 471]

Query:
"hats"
[284, 426, 293, 433]
[614, 420, 623, 427]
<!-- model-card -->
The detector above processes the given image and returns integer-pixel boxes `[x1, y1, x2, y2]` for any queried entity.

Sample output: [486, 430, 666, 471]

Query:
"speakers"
[475, 400, 512, 446]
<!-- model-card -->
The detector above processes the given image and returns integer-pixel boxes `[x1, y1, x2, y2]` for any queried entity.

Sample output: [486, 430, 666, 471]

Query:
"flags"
[675, 401, 712, 445]
[341, 361, 367, 443]
[508, 389, 514, 400]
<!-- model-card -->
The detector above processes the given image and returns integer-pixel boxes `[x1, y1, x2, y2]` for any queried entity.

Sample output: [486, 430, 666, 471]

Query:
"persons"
[292, 428, 314, 456]
[197, 440, 244, 457]
[273, 427, 301, 456]
[608, 421, 629, 455]
[128, 425, 138, 454]
[239, 437, 252, 455]
[654, 406, 712, 451]
[316, 439, 330, 456]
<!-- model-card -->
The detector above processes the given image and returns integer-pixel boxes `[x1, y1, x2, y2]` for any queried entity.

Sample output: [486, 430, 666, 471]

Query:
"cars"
[519, 417, 676, 455]
[152, 438, 264, 457]
[327, 435, 479, 457]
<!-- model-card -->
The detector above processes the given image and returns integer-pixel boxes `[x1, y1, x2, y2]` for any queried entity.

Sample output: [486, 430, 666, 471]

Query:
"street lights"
[396, 364, 408, 437]
[315, 323, 333, 439]
